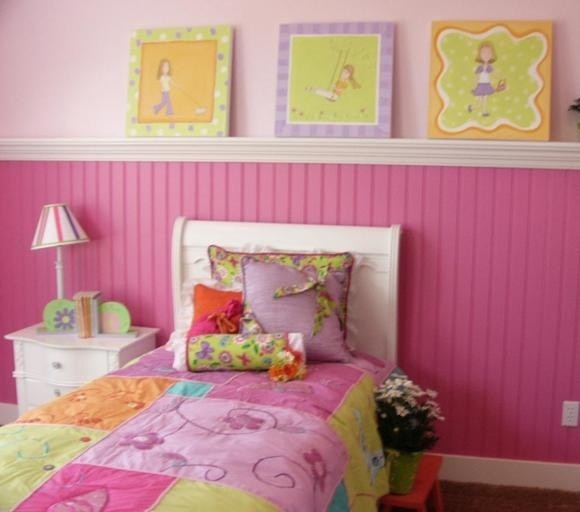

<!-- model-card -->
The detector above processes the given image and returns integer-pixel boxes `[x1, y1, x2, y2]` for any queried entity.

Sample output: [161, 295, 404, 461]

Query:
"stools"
[374, 454, 446, 509]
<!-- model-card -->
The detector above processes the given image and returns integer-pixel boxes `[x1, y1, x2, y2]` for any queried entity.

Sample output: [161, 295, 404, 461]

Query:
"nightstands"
[2, 319, 160, 424]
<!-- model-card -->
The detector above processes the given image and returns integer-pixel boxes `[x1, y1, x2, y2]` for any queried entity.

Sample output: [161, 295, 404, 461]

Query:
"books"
[73, 287, 103, 340]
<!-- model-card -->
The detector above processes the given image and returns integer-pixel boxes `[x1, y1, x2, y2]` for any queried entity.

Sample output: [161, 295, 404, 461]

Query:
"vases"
[379, 445, 423, 496]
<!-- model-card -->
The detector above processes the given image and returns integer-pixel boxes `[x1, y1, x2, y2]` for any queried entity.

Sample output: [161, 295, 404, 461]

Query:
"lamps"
[28, 200, 93, 335]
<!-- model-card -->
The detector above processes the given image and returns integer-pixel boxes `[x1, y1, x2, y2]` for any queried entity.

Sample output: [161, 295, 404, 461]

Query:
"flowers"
[368, 369, 444, 451]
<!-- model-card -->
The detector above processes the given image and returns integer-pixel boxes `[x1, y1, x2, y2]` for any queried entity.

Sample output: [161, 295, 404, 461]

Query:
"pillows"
[159, 236, 361, 386]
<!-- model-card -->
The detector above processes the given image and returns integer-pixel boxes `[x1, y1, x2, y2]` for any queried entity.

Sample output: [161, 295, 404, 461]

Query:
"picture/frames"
[270, 17, 398, 139]
[122, 21, 234, 140]
[423, 19, 558, 143]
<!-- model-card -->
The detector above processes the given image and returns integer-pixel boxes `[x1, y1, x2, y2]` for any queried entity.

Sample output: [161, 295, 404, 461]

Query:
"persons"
[463, 40, 502, 121]
[151, 57, 177, 117]
[300, 63, 362, 103]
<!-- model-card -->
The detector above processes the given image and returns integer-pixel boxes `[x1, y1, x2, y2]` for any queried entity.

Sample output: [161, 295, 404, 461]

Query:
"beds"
[0, 214, 411, 512]
